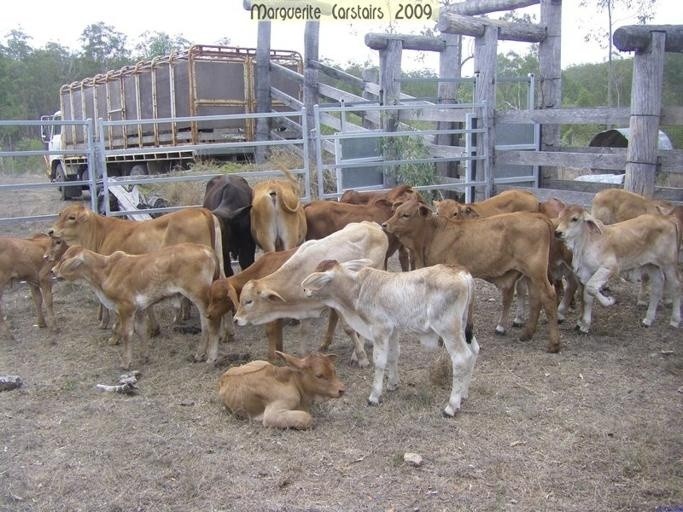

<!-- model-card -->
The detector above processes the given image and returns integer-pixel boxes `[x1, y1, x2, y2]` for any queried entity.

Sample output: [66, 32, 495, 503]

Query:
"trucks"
[37, 43, 310, 201]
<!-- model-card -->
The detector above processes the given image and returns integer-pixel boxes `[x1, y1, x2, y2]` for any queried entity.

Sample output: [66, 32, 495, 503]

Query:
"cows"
[214, 347, 349, 433]
[232, 220, 391, 369]
[0, 232, 62, 337]
[51, 242, 220, 371]
[589, 188, 683, 313]
[201, 236, 341, 359]
[303, 259, 482, 418]
[304, 185, 580, 337]
[554, 204, 683, 335]
[381, 199, 562, 355]
[203, 174, 256, 277]
[250, 177, 310, 253]
[47, 202, 238, 346]
